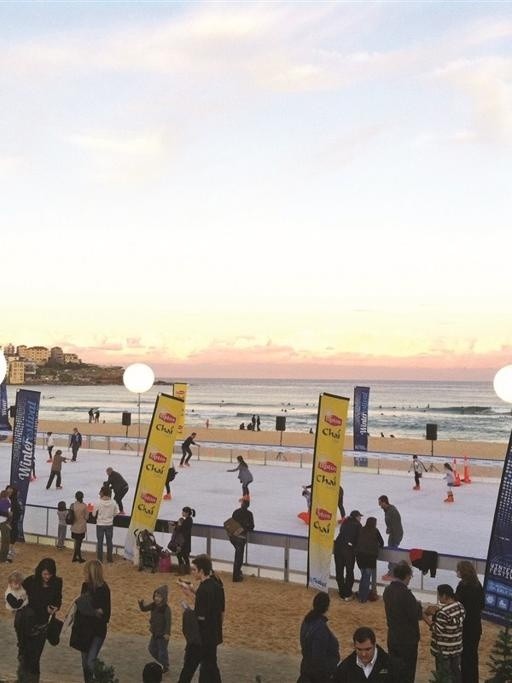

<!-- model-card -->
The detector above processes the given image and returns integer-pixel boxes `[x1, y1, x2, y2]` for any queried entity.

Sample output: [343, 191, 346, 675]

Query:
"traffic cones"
[452, 457, 461, 486]
[462, 456, 472, 483]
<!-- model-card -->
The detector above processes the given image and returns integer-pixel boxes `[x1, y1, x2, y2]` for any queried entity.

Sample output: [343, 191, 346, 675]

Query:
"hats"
[350, 510, 364, 517]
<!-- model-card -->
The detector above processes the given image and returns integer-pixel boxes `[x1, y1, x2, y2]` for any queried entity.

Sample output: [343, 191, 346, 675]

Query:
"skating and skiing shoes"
[444, 495, 454, 502]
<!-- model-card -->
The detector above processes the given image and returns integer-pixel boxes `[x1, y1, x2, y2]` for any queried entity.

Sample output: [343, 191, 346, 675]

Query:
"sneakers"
[164, 495, 172, 500]
[185, 462, 190, 466]
[179, 463, 184, 467]
[240, 497, 246, 500]
[245, 497, 250, 501]
[344, 594, 354, 602]
[56, 485, 61, 490]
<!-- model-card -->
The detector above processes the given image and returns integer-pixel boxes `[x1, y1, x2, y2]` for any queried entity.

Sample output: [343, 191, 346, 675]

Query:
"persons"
[390, 435, 394, 438]
[45, 450, 67, 490]
[252, 414, 256, 432]
[4, 485, 14, 500]
[333, 626, 395, 683]
[103, 468, 129, 513]
[60, 560, 111, 683]
[453, 561, 482, 683]
[137, 585, 171, 673]
[239, 423, 245, 430]
[378, 496, 403, 575]
[383, 561, 423, 683]
[309, 428, 313, 434]
[256, 415, 261, 431]
[247, 423, 253, 430]
[333, 511, 364, 599]
[297, 593, 341, 683]
[227, 456, 253, 502]
[13, 557, 63, 683]
[45, 432, 54, 462]
[224, 501, 255, 582]
[0, 490, 13, 563]
[92, 486, 119, 563]
[28, 455, 36, 480]
[164, 460, 175, 499]
[442, 463, 456, 502]
[408, 455, 427, 490]
[70, 492, 90, 563]
[302, 484, 346, 524]
[180, 433, 200, 468]
[355, 517, 384, 603]
[67, 428, 83, 461]
[302, 487, 311, 512]
[423, 585, 466, 683]
[8, 485, 22, 561]
[57, 502, 68, 550]
[142, 661, 163, 683]
[170, 507, 195, 576]
[381, 433, 384, 438]
[5, 572, 49, 621]
[88, 409, 93, 424]
[94, 410, 100, 424]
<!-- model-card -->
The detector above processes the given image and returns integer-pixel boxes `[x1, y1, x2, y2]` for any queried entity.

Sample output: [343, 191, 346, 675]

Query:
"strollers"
[134, 529, 163, 574]
